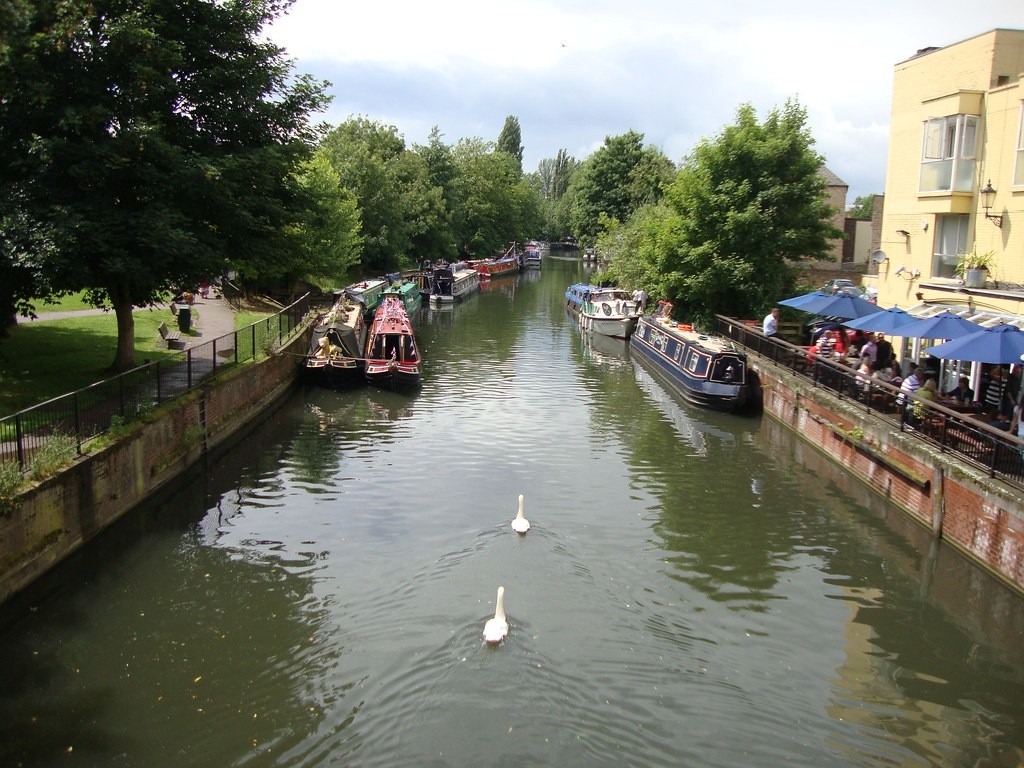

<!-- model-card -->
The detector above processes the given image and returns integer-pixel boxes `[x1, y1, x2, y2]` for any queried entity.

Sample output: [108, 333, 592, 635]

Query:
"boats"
[307, 260, 480, 393]
[632, 314, 750, 410]
[583, 247, 596, 261]
[466, 257, 520, 278]
[565, 282, 638, 338]
[523, 249, 543, 270]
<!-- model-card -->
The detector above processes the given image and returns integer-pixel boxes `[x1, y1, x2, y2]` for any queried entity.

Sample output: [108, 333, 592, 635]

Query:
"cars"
[839, 286, 877, 305]
[804, 315, 852, 337]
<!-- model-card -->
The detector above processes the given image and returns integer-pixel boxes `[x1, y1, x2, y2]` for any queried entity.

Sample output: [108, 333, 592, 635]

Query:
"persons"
[816, 326, 1024, 454]
[632, 287, 648, 314]
[199, 281, 212, 299]
[762, 308, 787, 342]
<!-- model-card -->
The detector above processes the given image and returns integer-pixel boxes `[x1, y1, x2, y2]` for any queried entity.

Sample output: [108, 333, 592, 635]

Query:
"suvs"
[824, 278, 854, 296]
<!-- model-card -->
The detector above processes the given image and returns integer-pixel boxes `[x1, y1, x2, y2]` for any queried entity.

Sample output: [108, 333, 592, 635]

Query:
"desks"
[833, 356, 862, 366]
[961, 412, 1018, 445]
[935, 395, 972, 414]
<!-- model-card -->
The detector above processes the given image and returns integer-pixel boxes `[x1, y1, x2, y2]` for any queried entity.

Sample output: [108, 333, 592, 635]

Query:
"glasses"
[878, 337, 884, 339]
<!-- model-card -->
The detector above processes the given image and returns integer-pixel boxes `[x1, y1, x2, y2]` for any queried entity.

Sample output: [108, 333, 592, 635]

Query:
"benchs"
[158, 322, 181, 348]
[170, 301, 179, 316]
[794, 345, 994, 459]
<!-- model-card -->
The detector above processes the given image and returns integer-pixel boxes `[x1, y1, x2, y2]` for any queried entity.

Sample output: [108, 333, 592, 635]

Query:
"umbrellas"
[808, 291, 885, 320]
[778, 288, 833, 347]
[924, 320, 1024, 415]
[885, 310, 989, 394]
[839, 304, 921, 367]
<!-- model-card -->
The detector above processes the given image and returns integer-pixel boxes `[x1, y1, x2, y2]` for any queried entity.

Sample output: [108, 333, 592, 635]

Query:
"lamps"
[896, 229, 909, 238]
[980, 178, 1003, 228]
[893, 264, 919, 280]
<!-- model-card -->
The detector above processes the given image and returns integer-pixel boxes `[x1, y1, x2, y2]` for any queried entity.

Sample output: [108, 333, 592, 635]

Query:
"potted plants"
[953, 243, 1000, 289]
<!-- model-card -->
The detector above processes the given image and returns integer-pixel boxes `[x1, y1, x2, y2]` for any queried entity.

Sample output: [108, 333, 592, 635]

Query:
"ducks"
[483, 586, 509, 643]
[511, 495, 530, 533]
